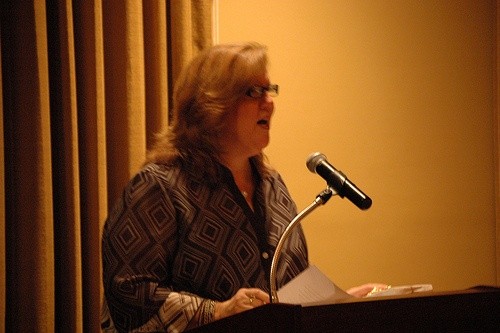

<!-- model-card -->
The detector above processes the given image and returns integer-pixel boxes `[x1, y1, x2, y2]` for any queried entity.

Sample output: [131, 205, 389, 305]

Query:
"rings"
[248, 295, 255, 306]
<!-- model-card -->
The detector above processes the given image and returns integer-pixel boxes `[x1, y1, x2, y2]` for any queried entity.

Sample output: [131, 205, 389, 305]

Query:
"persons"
[100, 40, 390, 332]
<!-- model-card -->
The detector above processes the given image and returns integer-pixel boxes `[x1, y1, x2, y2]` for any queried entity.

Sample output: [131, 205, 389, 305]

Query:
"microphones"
[306, 154, 372, 212]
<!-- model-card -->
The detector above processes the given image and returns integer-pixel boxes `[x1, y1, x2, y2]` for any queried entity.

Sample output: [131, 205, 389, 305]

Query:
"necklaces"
[240, 189, 250, 199]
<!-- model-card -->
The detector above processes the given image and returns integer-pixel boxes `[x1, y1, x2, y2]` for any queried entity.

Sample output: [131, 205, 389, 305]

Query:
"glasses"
[243, 83, 279, 99]
